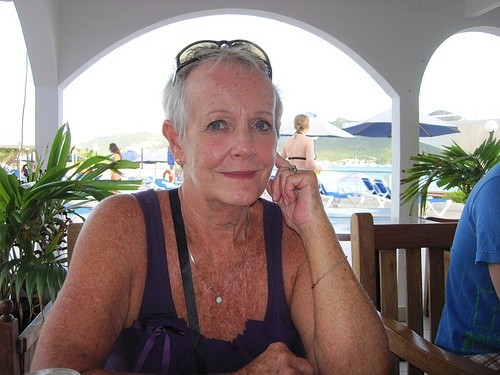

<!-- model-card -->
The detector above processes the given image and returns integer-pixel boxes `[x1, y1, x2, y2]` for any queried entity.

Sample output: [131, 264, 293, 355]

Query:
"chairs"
[350, 213, 500, 375]
[315, 177, 454, 216]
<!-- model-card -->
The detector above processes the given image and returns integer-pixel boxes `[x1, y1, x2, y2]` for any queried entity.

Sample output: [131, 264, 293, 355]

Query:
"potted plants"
[0, 121, 142, 375]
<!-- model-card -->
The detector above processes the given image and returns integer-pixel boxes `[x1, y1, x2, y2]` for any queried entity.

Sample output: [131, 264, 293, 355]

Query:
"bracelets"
[312, 255, 347, 288]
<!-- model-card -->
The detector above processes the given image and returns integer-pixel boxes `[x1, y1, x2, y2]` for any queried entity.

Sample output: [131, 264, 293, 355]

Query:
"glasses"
[171, 39, 272, 86]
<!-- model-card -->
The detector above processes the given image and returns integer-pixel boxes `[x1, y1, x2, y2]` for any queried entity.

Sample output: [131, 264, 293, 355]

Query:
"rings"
[281, 165, 298, 174]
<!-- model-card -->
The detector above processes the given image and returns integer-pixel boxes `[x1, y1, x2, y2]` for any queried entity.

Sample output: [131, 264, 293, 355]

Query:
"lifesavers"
[162, 170, 173, 182]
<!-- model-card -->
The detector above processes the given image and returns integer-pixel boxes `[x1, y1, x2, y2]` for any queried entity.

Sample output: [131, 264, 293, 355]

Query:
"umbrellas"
[342, 109, 462, 139]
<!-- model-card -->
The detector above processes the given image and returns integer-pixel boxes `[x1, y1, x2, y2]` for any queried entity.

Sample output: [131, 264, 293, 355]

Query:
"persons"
[108, 143, 121, 195]
[425, 163, 500, 375]
[280, 114, 318, 171]
[28, 39, 392, 375]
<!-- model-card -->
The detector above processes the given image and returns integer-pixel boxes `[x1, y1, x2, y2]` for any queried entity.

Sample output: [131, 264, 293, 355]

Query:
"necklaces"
[185, 205, 251, 304]
